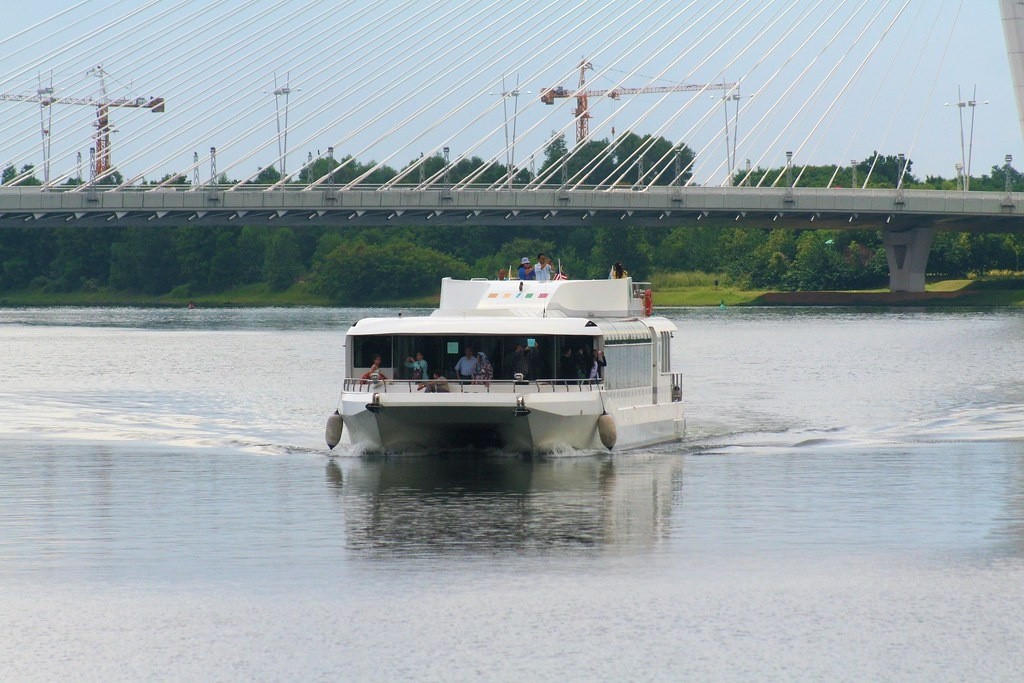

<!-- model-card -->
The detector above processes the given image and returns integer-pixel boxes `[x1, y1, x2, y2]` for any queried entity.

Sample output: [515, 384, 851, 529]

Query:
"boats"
[325, 253, 684, 451]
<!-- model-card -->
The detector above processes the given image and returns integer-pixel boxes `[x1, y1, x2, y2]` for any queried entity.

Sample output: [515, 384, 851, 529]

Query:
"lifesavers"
[360, 372, 386, 384]
[645, 288, 652, 316]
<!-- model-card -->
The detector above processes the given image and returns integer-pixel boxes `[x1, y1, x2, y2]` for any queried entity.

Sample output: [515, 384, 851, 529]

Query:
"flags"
[553, 262, 568, 280]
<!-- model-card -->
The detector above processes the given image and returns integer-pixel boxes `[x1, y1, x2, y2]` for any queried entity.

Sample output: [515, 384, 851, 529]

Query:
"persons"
[508, 341, 538, 386]
[495, 267, 509, 281]
[612, 262, 628, 280]
[517, 257, 536, 281]
[369, 352, 382, 375]
[417, 370, 449, 393]
[405, 350, 429, 384]
[455, 345, 479, 385]
[470, 351, 493, 385]
[557, 343, 606, 387]
[534, 253, 556, 280]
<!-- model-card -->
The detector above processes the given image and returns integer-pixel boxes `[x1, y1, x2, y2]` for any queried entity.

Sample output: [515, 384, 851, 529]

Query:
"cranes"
[0, 62, 165, 187]
[540, 54, 741, 147]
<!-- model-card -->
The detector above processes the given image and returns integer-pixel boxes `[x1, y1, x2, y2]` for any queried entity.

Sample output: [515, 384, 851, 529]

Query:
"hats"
[520, 257, 530, 264]
